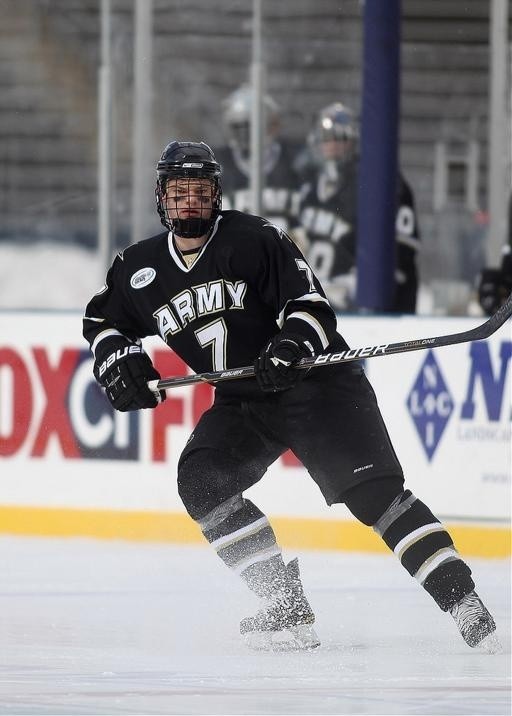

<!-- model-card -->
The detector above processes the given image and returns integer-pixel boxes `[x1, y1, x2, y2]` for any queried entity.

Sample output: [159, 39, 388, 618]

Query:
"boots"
[449, 590, 496, 648]
[240, 557, 314, 634]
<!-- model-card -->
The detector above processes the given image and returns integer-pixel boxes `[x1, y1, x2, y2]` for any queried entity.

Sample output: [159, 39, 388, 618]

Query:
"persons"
[208, 81, 319, 234]
[292, 100, 422, 315]
[79, 141, 498, 651]
[457, 208, 493, 283]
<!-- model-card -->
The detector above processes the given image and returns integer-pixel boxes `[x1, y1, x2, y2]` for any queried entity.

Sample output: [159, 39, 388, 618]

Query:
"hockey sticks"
[147, 288, 510, 390]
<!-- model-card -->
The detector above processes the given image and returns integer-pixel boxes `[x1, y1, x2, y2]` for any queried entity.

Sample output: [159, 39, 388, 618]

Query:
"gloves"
[94, 334, 167, 411]
[255, 330, 313, 393]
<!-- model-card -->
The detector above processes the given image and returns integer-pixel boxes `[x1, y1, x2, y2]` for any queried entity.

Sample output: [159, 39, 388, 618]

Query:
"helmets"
[224, 82, 281, 158]
[155, 140, 222, 238]
[307, 103, 361, 174]
